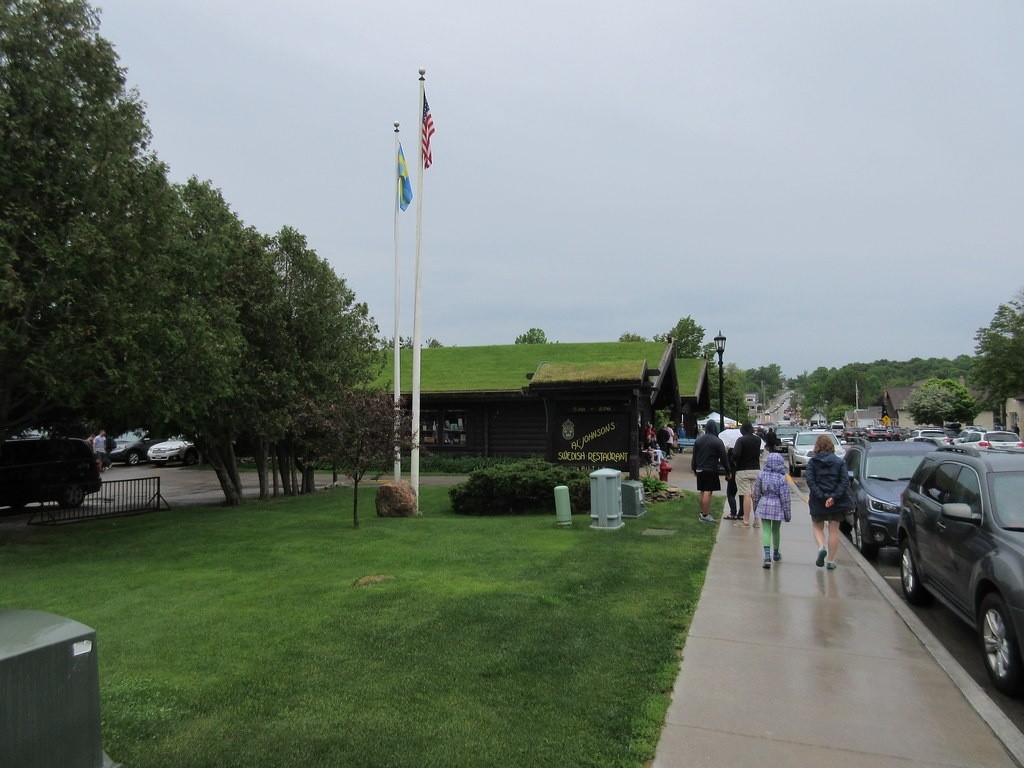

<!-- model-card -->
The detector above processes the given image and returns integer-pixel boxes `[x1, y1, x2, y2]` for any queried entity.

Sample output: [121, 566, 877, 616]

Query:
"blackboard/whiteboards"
[552, 400, 632, 466]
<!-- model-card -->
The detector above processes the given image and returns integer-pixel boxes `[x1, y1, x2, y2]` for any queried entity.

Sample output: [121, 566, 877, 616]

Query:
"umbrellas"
[719, 429, 766, 451]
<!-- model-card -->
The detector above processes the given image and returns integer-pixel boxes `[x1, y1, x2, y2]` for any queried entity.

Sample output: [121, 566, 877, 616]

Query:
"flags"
[422, 95, 435, 168]
[398, 145, 413, 212]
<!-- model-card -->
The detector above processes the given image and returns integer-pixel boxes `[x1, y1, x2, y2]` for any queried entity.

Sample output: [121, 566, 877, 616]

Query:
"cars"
[146, 433, 202, 467]
[757, 406, 1024, 479]
[106, 425, 178, 466]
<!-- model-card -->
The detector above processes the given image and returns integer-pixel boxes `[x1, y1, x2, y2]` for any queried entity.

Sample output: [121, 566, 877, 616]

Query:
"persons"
[692, 420, 761, 527]
[805, 434, 849, 568]
[753, 454, 792, 568]
[757, 427, 777, 453]
[88, 430, 112, 472]
[1014, 424, 1020, 436]
[640, 423, 696, 470]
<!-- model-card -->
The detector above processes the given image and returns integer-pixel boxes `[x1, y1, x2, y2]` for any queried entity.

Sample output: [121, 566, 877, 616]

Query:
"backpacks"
[107, 436, 116, 449]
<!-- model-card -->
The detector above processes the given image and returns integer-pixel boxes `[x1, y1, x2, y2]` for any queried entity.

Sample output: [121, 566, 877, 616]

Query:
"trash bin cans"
[590, 468, 625, 530]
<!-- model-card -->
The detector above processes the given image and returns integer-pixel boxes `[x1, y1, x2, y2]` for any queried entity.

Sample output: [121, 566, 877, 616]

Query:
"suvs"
[836, 437, 951, 561]
[895, 443, 1024, 697]
[0, 431, 103, 513]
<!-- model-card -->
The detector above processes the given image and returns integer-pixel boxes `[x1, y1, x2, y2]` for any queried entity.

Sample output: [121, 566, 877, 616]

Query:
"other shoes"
[723, 514, 738, 520]
[97, 464, 112, 473]
[753, 522, 760, 528]
[733, 521, 751, 528]
[737, 515, 744, 520]
[773, 552, 781, 561]
[642, 453, 676, 470]
[698, 513, 718, 523]
[827, 563, 836, 569]
[816, 546, 827, 567]
[763, 558, 771, 569]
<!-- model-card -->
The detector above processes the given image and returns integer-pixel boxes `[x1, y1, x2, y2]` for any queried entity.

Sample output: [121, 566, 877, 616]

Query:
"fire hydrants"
[657, 461, 673, 483]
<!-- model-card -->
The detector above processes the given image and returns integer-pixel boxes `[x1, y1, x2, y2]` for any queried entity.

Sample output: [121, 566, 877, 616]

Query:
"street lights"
[714, 331, 727, 432]
